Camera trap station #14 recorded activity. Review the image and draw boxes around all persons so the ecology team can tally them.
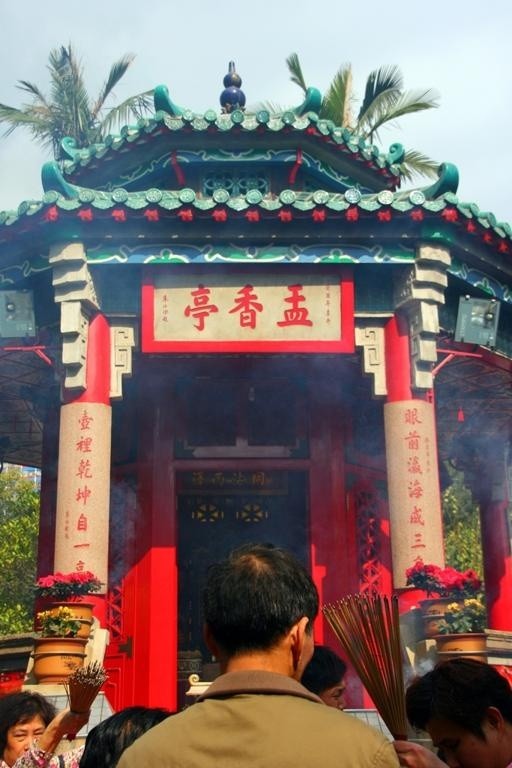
[115,538,399,768]
[300,645,349,713]
[1,689,93,768]
[391,659,511,768]
[78,706,176,767]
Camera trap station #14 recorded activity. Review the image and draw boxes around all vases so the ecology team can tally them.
[44,603,95,639]
[31,637,88,686]
[417,598,464,639]
[433,633,488,666]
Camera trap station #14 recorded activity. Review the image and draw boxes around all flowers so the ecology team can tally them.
[437,598,488,634]
[37,605,83,638]
[33,571,106,602]
[405,561,481,602]
